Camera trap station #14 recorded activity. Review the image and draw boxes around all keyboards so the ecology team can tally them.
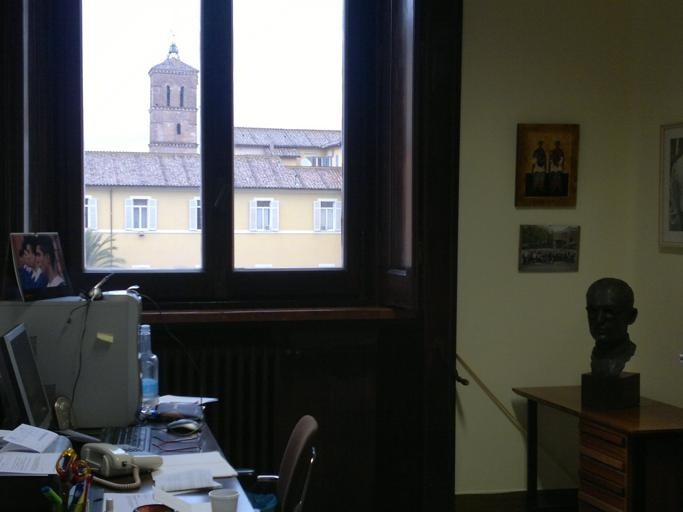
[108,425,153,453]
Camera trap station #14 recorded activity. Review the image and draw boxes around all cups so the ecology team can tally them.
[208,488,241,512]
[133,503,174,511]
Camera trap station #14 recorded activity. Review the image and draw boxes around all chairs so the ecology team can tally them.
[230,411,322,511]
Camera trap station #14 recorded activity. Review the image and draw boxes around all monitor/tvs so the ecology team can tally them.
[2,323,54,429]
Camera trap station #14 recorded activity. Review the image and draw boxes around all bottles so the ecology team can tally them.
[136,324,159,415]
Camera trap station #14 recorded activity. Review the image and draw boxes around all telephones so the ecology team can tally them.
[79,440,164,479]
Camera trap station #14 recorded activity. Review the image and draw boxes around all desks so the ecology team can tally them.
[73,403,259,511]
[508,382,682,512]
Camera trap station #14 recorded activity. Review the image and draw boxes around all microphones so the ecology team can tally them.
[65,298,92,324]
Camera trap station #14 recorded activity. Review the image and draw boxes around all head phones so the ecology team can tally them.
[87,274,118,301]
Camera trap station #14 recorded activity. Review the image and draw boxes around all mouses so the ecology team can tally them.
[167,418,200,435]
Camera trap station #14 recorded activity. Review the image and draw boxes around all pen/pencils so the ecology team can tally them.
[41,474,90,512]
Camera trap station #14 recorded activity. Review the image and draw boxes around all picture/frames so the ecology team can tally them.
[512,121,581,211]
[655,119,682,255]
[8,230,74,304]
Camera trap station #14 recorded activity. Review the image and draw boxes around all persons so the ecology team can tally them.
[17,235,67,289]
[586,277,638,378]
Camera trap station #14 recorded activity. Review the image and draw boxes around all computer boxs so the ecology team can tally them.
[0,289,147,429]
